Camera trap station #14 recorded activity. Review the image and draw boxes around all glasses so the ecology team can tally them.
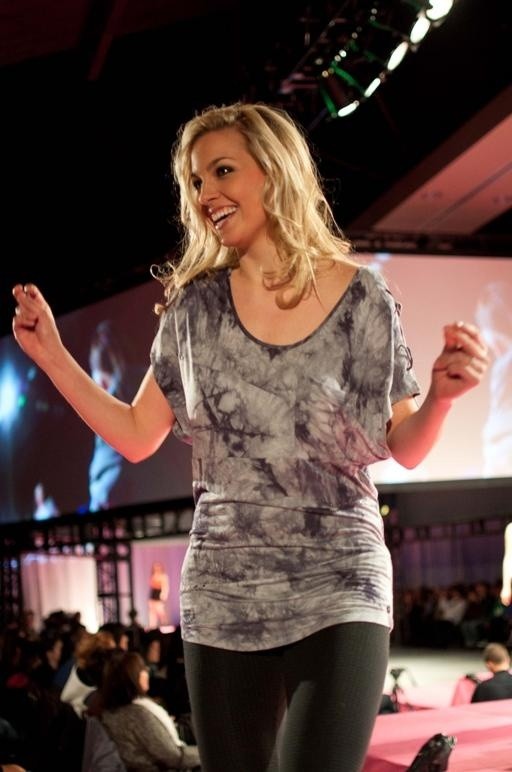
[279,0,454,120]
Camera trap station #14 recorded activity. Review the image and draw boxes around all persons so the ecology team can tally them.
[148,562,169,629]
[471,643,510,703]
[2,102,495,771]
[392,581,510,651]
[1,609,204,770]
